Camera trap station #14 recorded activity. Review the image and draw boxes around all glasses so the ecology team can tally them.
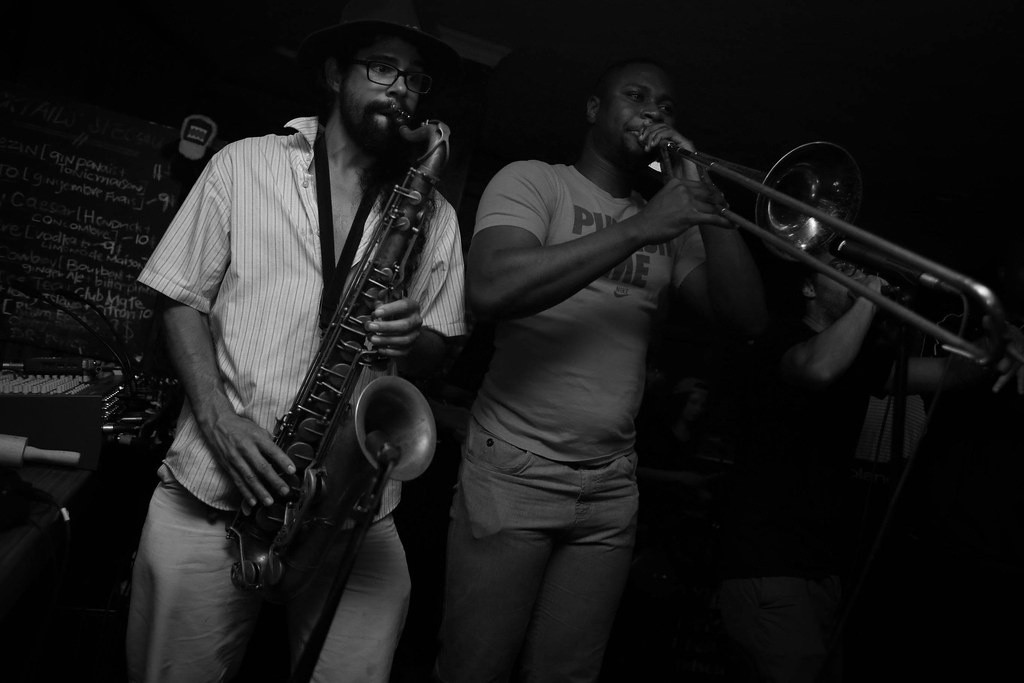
[339,57,432,95]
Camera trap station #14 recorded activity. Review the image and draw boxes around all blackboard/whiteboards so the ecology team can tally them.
[0,82,229,370]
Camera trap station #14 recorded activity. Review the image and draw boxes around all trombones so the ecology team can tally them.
[660,140,1005,369]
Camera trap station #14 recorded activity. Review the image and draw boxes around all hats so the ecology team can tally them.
[296,0,462,100]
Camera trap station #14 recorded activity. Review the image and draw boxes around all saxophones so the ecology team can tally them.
[225,108,457,613]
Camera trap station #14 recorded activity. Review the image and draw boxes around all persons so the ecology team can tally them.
[422,50,767,683]
[650,377,709,456]
[124,0,469,683]
[713,244,1024,683]
